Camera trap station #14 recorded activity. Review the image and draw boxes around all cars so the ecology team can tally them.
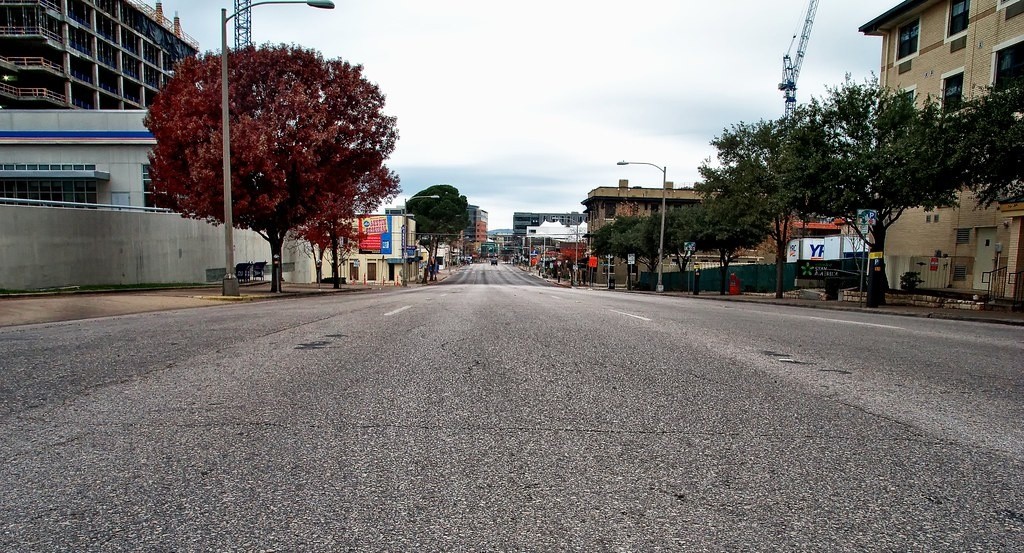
[491,259,497,265]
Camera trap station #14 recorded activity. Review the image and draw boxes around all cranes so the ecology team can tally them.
[778,0,820,121]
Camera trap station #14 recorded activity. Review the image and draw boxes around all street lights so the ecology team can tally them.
[404,195,440,278]
[220,0,336,297]
[617,159,664,292]
[552,217,578,286]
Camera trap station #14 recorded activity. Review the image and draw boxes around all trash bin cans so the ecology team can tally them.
[729,279,740,295]
[610,279,615,289]
[429,264,439,275]
